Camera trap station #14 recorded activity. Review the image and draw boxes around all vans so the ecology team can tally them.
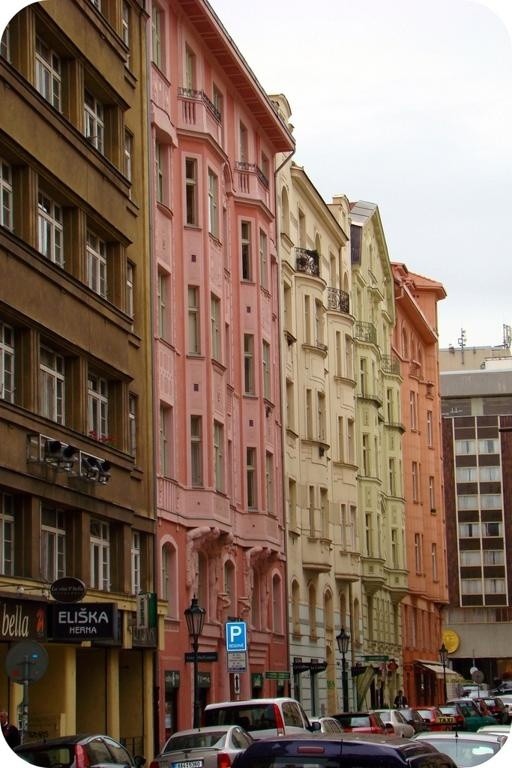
[203,696,321,740]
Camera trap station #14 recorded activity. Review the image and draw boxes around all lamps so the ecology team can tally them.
[71,450,111,485]
[26,432,78,474]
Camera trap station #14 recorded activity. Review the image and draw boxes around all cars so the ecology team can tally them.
[16,734,146,768]
[313,693,511,739]
[148,725,252,768]
[232,725,509,767]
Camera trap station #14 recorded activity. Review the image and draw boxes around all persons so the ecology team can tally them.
[0,711,18,748]
[393,690,408,709]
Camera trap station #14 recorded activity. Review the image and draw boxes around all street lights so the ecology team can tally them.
[185,593,206,729]
[337,625,351,712]
[440,642,449,704]
